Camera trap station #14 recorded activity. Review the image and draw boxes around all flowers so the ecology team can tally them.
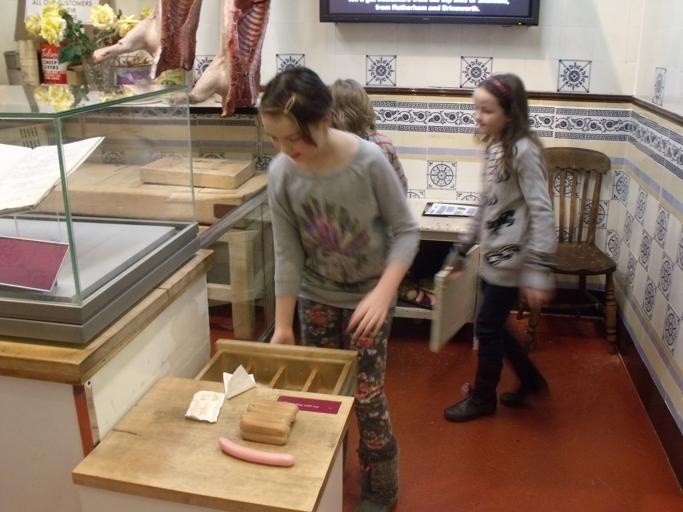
[21,0,158,68]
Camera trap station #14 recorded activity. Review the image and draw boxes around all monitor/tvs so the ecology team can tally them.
[320,0,540,26]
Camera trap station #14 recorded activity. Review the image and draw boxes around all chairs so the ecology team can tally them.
[515,145,620,356]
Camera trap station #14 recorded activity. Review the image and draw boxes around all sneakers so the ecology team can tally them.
[354,496,390,512]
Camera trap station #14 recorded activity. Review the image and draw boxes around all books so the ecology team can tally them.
[427,241,481,356]
[0,134,109,223]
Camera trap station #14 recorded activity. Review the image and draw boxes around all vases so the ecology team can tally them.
[80,53,118,87]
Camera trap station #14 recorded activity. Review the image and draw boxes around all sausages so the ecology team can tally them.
[240,400,298,445]
[220,437,293,467]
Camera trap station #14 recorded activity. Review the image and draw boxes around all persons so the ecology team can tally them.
[256,64,427,512]
[322,78,407,206]
[440,68,563,424]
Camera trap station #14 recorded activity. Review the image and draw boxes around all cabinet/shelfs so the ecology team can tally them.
[0,82,198,324]
[390,198,482,351]
[70,337,364,510]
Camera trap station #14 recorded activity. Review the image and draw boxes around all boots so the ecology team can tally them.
[500,362,549,410]
[443,376,500,424]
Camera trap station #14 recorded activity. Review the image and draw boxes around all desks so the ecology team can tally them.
[0,248,221,511]
[195,208,275,340]
